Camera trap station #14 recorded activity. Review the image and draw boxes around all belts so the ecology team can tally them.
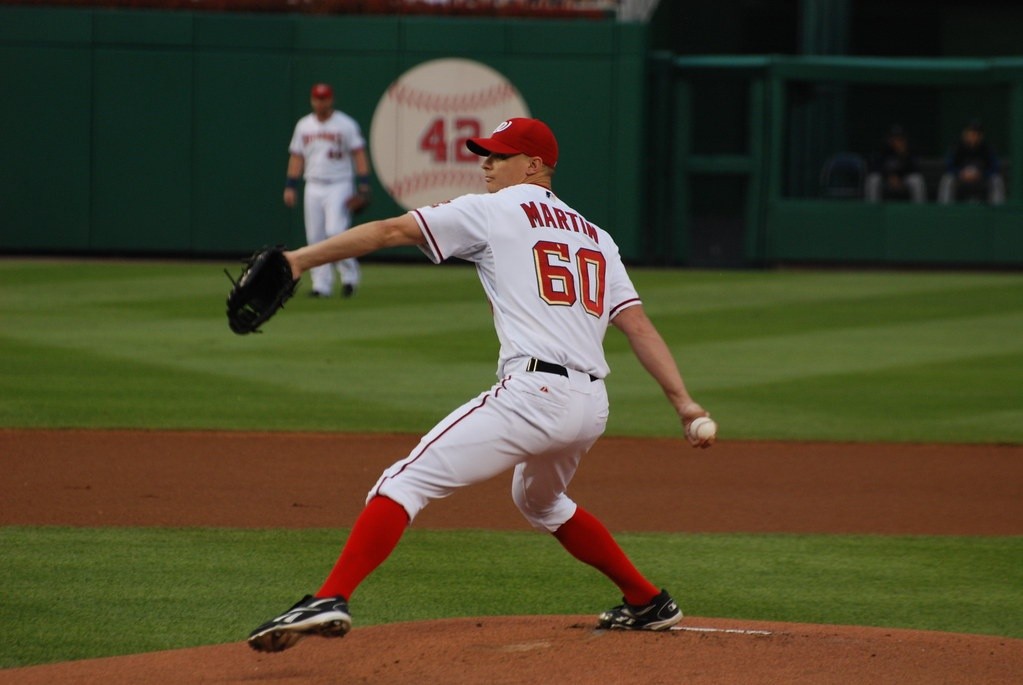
[526,358,599,382]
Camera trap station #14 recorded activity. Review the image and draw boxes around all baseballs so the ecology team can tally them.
[687,415,718,442]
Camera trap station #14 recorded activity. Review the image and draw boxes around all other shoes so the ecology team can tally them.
[308,291,319,298]
[341,284,352,298]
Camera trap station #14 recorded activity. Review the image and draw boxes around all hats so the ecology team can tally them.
[311,83,331,98]
[466,117,559,169]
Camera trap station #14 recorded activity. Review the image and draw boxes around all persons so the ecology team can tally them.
[864,131,924,205]
[283,83,373,299]
[939,126,1006,207]
[226,118,715,655]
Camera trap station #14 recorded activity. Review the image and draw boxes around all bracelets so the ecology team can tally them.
[357,177,369,183]
[284,177,295,188]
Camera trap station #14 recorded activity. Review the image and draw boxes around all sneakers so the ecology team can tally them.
[248,595,351,653]
[599,589,684,631]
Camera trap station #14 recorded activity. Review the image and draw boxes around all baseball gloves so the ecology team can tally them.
[226,242,302,336]
